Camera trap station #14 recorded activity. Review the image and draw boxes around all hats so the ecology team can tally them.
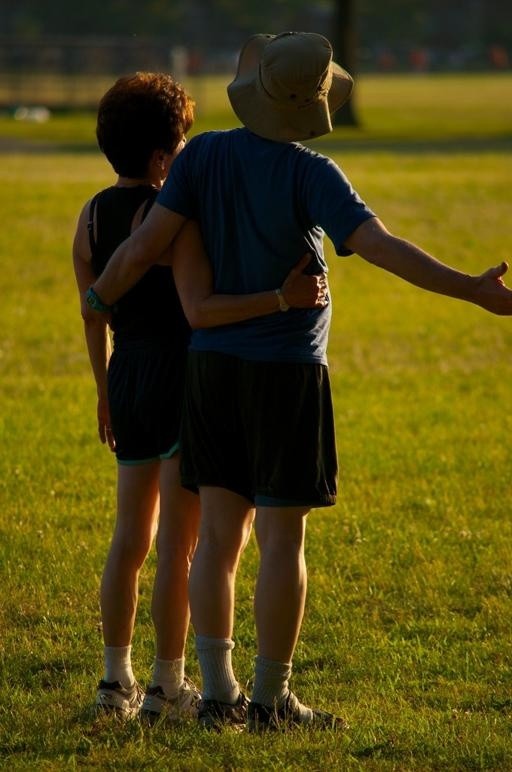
[228,32,354,141]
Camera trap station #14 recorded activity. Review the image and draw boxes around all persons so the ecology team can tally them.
[73,73,327,730]
[82,32,512,732]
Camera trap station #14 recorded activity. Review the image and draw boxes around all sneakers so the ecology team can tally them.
[96,676,345,734]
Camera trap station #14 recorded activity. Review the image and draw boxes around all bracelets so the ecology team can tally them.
[85,287,113,312]
[275,287,290,313]
